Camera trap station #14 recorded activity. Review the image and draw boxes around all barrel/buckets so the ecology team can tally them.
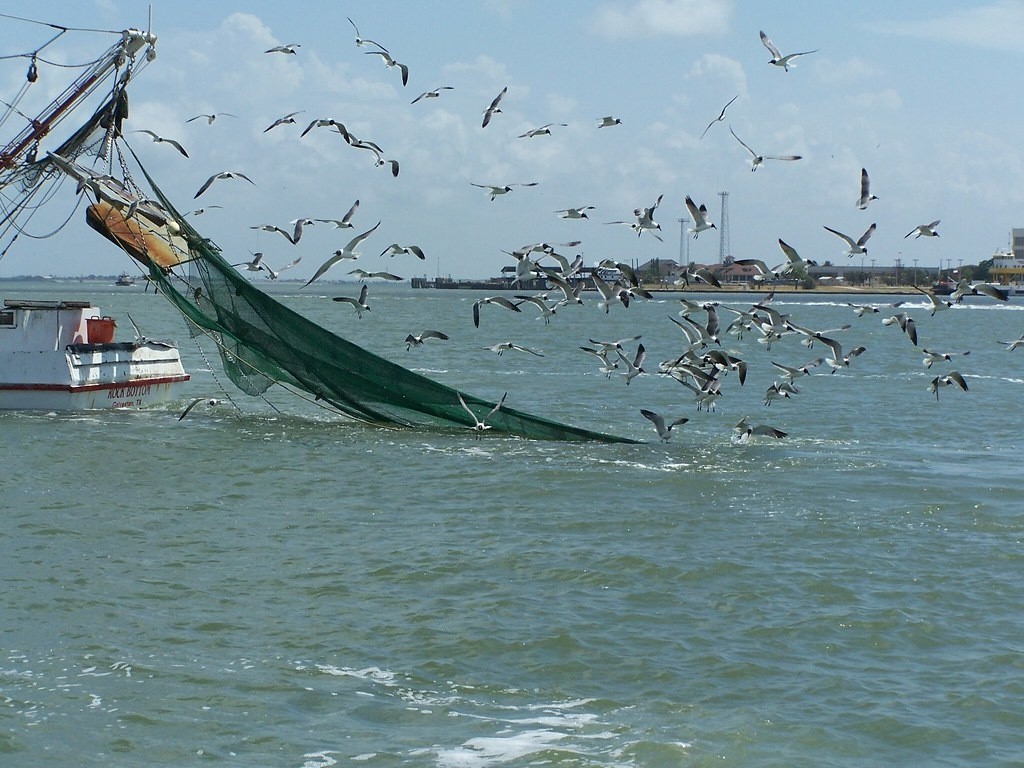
[86,316,116,343]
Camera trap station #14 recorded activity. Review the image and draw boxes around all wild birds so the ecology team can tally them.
[124,15,1024,446]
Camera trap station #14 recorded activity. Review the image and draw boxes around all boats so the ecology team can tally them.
[489,266,633,291]
[115,275,134,286]
[0,299,190,409]
